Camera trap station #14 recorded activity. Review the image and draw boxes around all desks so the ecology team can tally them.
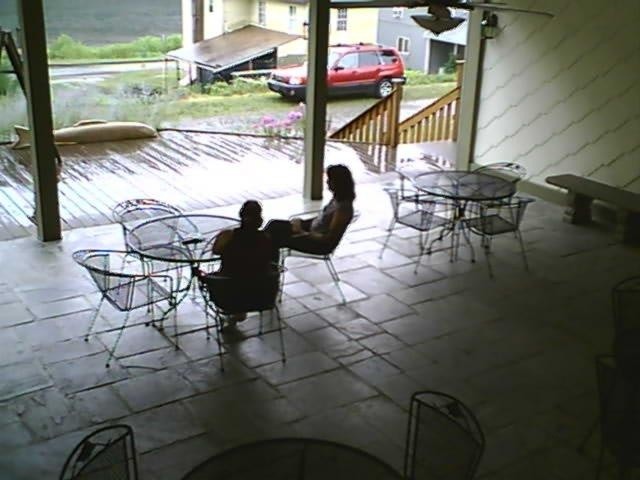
[127,212,242,340]
[182,437,404,480]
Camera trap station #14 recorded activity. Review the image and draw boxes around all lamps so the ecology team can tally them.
[411,4,467,36]
[481,14,501,40]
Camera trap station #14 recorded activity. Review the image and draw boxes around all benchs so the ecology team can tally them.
[545,174,640,242]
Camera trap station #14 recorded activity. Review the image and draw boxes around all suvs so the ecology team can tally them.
[268,41,407,100]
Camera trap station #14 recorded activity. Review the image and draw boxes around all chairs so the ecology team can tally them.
[577,277,640,480]
[59,423,138,479]
[73,249,179,370]
[380,162,536,277]
[112,198,204,328]
[276,209,360,303]
[195,264,289,371]
[404,390,486,480]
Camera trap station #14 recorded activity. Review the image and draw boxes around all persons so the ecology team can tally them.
[262,163,357,256]
[211,199,273,323]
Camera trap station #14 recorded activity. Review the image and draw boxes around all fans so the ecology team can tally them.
[333,0,555,17]
